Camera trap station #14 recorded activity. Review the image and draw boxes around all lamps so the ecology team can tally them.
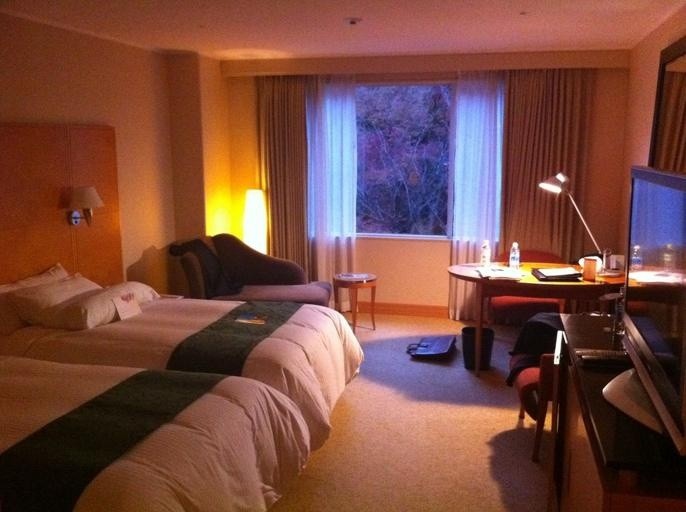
[537,172,603,255]
[67,187,104,227]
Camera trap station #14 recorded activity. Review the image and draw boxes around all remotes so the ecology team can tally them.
[575,349,629,362]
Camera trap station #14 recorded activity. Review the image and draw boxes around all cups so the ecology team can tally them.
[583,258,597,283]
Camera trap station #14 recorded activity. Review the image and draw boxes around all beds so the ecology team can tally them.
[1,355,310,511]
[1,291,365,453]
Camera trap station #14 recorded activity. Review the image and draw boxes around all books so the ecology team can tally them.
[538,266,581,277]
[477,264,522,282]
[531,267,581,282]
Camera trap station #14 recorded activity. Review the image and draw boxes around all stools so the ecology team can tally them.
[333,272,376,336]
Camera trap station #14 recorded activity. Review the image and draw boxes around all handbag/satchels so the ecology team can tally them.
[407,335,456,358]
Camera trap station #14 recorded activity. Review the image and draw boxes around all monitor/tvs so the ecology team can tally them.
[600,163,686,459]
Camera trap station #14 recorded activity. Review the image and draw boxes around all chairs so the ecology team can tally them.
[167,233,332,308]
[508,311,560,463]
[486,250,568,322]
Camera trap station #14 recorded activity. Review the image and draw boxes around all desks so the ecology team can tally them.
[446,261,626,381]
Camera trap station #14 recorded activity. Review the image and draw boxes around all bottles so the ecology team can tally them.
[613,287,627,337]
[662,244,675,273]
[480,239,491,271]
[508,242,520,272]
[630,245,644,272]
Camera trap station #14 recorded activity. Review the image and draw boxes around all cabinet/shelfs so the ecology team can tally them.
[559,313,684,511]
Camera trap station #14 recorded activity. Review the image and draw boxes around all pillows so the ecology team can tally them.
[0,262,160,339]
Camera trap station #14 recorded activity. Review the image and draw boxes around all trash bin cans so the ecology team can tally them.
[462,327,494,370]
